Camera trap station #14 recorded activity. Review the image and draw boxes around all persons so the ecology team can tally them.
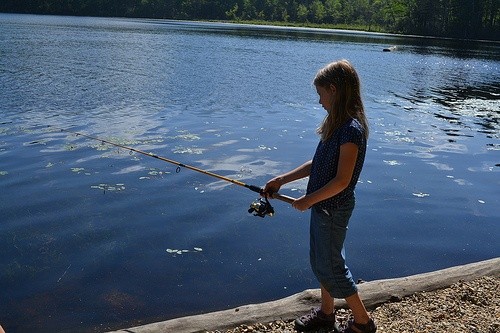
[260,57,382,333]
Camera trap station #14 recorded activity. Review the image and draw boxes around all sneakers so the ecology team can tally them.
[343,318,376,333]
[295,307,335,330]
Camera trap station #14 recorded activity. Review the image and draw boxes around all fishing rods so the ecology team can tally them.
[34,123,312,211]
